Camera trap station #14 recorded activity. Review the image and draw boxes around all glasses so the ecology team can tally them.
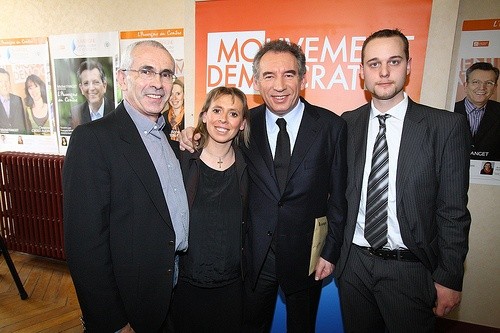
[82,81,102,88]
[467,80,495,87]
[121,68,178,84]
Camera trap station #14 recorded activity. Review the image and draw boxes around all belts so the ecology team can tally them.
[364,246,421,262]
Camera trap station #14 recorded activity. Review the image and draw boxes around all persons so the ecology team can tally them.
[161,79,185,141]
[24,74,55,135]
[0,68,26,134]
[62,137,67,146]
[180,41,347,333]
[174,87,248,333]
[18,136,23,144]
[454,62,500,161]
[334,29,471,333]
[1,135,5,142]
[70,60,115,130]
[60,41,192,333]
[480,162,493,175]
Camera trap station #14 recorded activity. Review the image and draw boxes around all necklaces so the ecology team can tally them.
[217,158,223,168]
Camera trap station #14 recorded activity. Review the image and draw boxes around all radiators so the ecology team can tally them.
[0,151,67,261]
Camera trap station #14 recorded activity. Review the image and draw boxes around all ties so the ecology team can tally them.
[364,114,392,250]
[274,118,291,197]
